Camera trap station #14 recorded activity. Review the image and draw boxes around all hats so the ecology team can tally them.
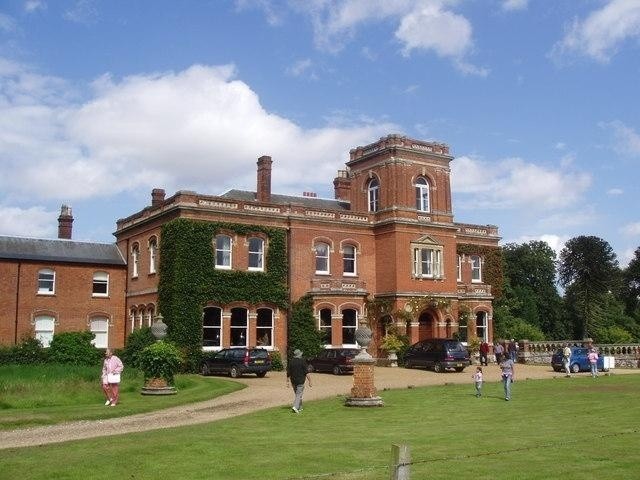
[292,348,304,359]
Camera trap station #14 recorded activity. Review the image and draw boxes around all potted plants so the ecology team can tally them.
[379,334,404,368]
[470,336,482,367]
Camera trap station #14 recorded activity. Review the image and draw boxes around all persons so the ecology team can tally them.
[562,342,571,378]
[287,349,312,413]
[588,346,599,379]
[101,349,124,406]
[473,338,519,401]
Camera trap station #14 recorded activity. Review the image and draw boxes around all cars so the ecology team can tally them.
[404,338,471,374]
[306,348,362,374]
[549,348,609,376]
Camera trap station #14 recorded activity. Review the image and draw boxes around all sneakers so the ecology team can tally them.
[110,403,116,407]
[104,398,111,406]
[292,406,304,414]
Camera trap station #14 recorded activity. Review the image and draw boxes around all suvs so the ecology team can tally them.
[200,347,271,378]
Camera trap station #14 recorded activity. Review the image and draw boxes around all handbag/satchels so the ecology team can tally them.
[106,370,121,384]
